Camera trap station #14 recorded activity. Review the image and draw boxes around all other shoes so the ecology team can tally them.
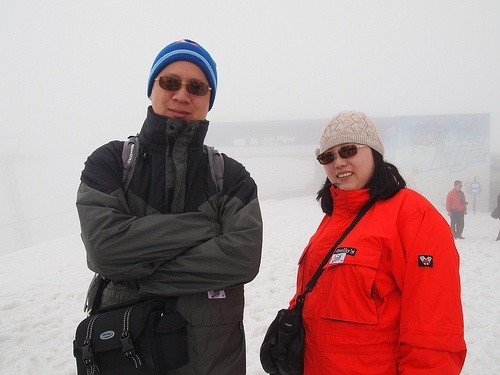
[454,235,465,239]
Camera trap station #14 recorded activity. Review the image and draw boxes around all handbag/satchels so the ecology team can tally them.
[260,308,305,375]
[72,295,189,375]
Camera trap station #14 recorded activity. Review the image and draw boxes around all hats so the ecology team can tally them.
[147,38,217,112]
[314,110,384,157]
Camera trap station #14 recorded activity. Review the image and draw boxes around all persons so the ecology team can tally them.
[446,181,467,239]
[288,111,466,375]
[77,39,262,375]
[496,193,500,240]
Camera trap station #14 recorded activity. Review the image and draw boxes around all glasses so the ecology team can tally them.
[316,144,368,165]
[154,75,212,96]
